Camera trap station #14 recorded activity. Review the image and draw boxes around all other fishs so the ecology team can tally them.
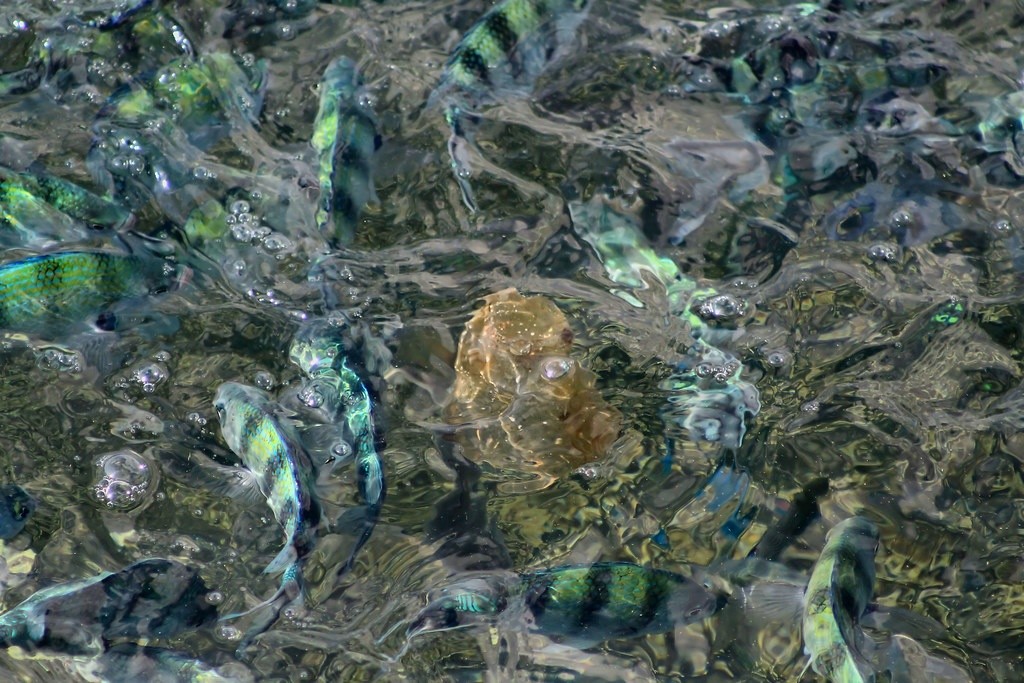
[0,1,1024,683]
